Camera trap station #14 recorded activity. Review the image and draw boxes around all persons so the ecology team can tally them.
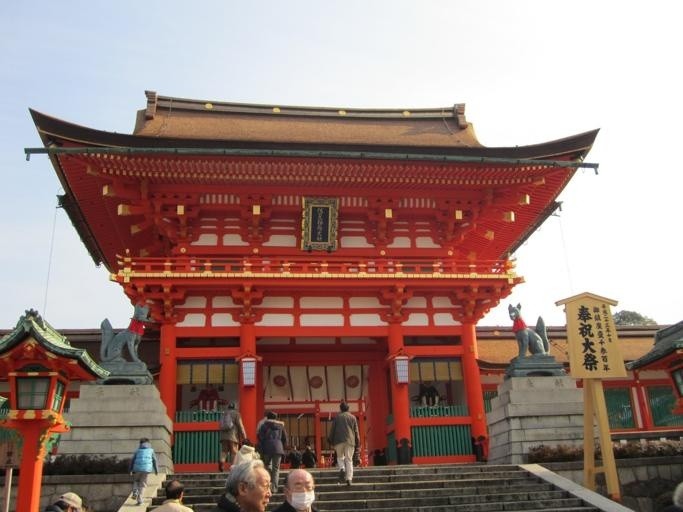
[211,461,316,512]
[218,401,286,493]
[150,480,194,512]
[41,491,82,512]
[328,401,358,486]
[287,445,317,470]
[128,437,159,503]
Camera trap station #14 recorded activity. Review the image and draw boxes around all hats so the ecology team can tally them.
[59,492,82,512]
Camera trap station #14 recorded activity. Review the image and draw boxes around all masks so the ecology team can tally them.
[287,490,316,510]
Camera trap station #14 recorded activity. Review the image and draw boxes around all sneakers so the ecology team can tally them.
[131,491,144,506]
[219,460,224,472]
[339,470,352,486]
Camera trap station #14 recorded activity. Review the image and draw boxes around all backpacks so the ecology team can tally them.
[219,410,234,432]
